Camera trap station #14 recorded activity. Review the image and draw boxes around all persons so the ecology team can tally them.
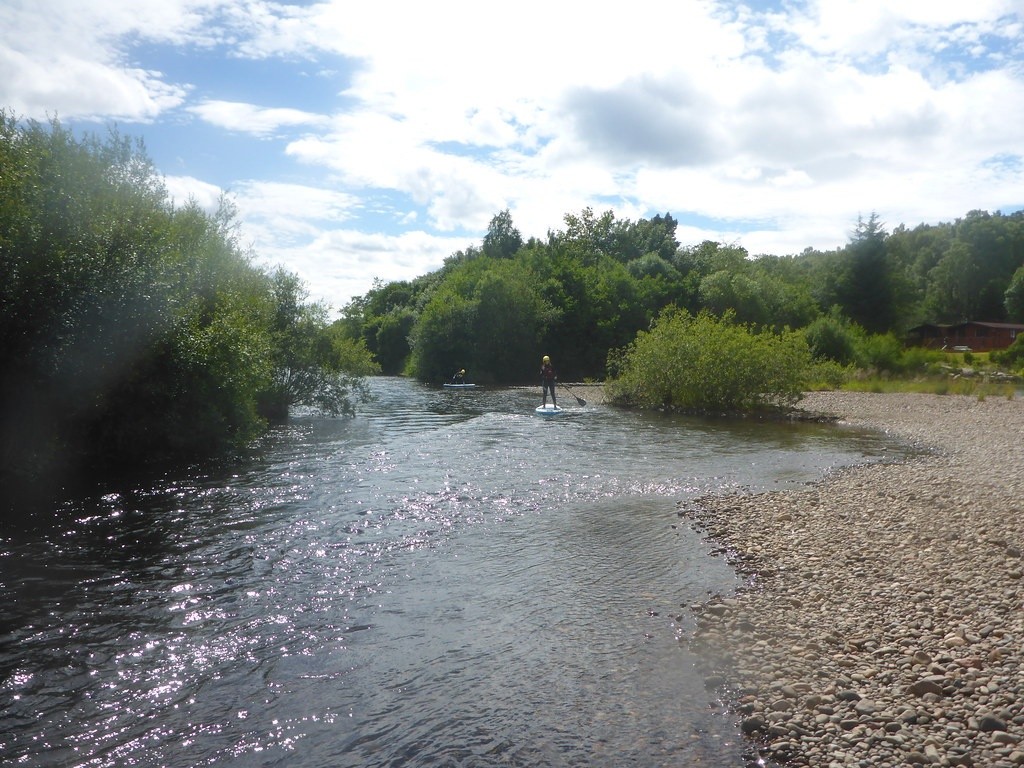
[450,368,466,384]
[538,356,561,410]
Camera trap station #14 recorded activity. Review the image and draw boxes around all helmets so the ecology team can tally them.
[543,356,550,363]
[461,370,465,374]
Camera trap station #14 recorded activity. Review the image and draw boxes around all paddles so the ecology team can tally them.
[557,379,586,407]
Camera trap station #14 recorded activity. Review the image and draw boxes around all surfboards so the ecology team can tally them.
[536,404,562,415]
[444,384,475,387]
